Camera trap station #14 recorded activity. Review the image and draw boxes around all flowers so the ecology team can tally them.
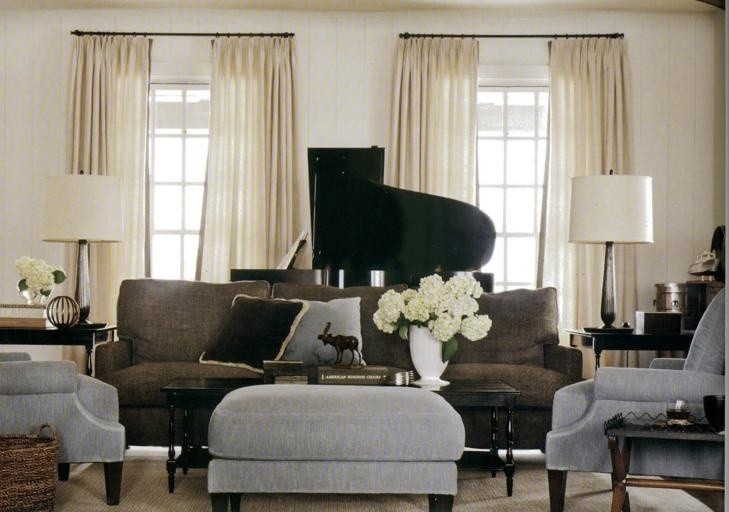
[14,255,67,297]
[373,274,493,363]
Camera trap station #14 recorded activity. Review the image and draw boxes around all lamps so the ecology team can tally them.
[39,170,124,329]
[568,169,654,332]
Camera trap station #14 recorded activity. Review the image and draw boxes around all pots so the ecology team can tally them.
[653,283,688,313]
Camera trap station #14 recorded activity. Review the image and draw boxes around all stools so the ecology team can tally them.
[207,383,465,512]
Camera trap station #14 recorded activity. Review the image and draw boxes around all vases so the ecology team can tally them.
[21,291,47,305]
[409,325,450,385]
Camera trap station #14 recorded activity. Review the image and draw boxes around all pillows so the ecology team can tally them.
[284,297,367,367]
[199,293,309,374]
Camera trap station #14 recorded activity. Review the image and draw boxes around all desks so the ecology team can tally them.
[0,318,117,375]
[565,325,695,369]
[605,417,725,512]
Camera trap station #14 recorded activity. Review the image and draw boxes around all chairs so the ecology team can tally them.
[0,351,125,506]
[544,285,725,511]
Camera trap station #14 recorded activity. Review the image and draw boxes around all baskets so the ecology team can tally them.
[0,424,61,512]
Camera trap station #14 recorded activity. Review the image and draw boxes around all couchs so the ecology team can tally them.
[94,279,583,454]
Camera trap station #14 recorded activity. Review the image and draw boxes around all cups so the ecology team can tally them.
[703,395,725,430]
[667,397,691,426]
